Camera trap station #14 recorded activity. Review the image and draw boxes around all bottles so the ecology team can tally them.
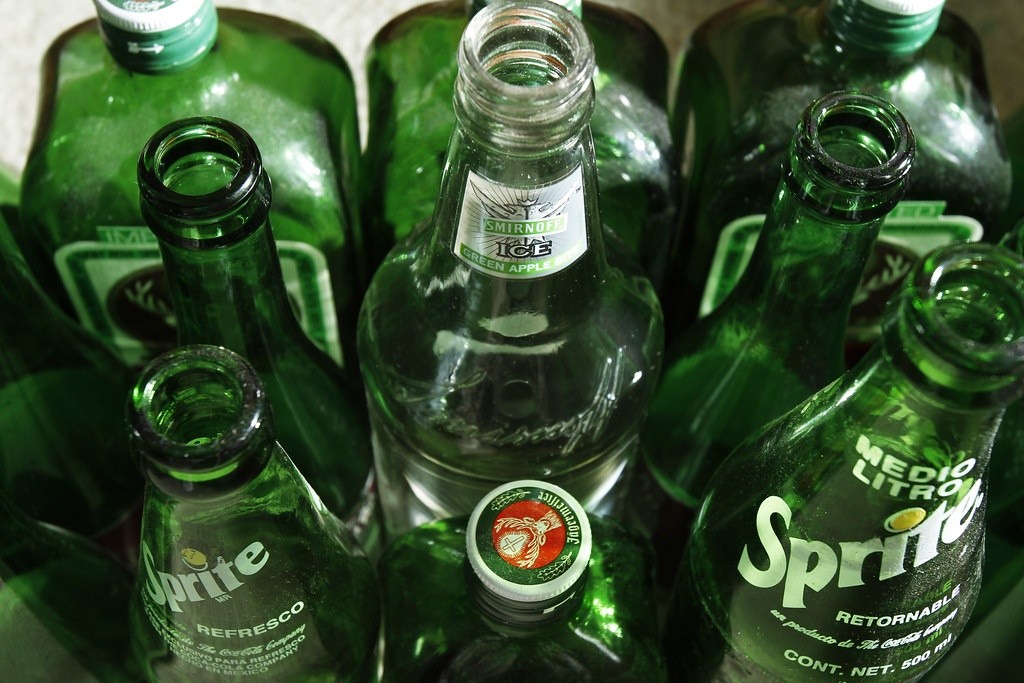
[673,0,1012,367]
[357,0,678,293]
[20,0,362,382]
[0,497,124,683]
[382,479,668,683]
[0,229,137,574]
[362,0,664,536]
[622,90,914,629]
[670,244,1024,683]
[125,345,378,683]
[138,118,385,561]
